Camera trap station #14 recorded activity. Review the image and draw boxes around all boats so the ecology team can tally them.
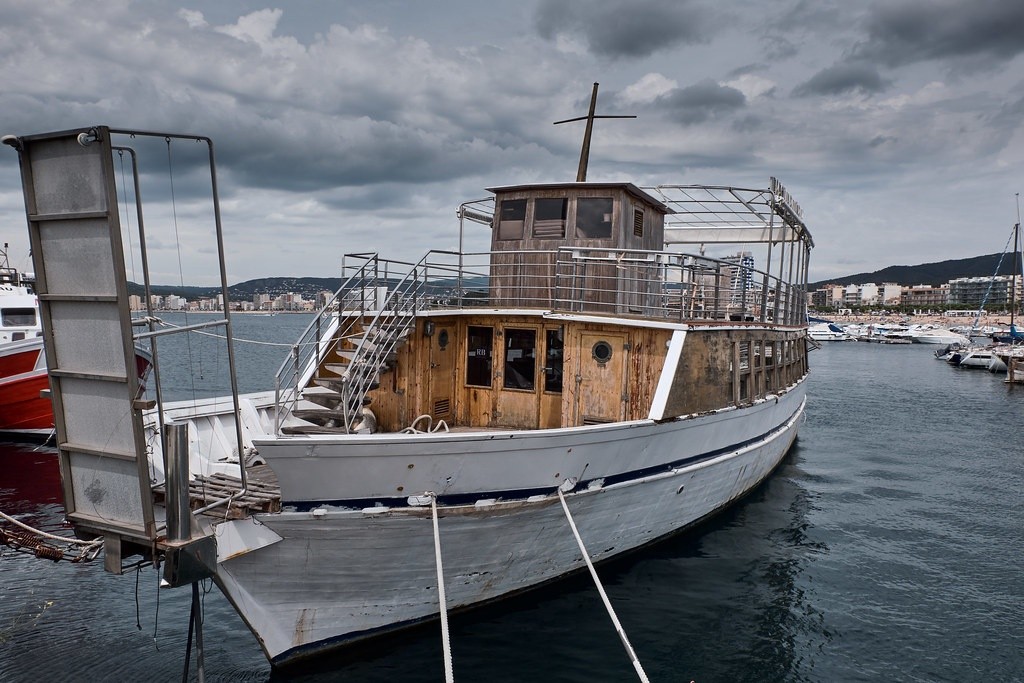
[0,244,155,437]
[169,82,815,670]
[806,191,1024,384]
